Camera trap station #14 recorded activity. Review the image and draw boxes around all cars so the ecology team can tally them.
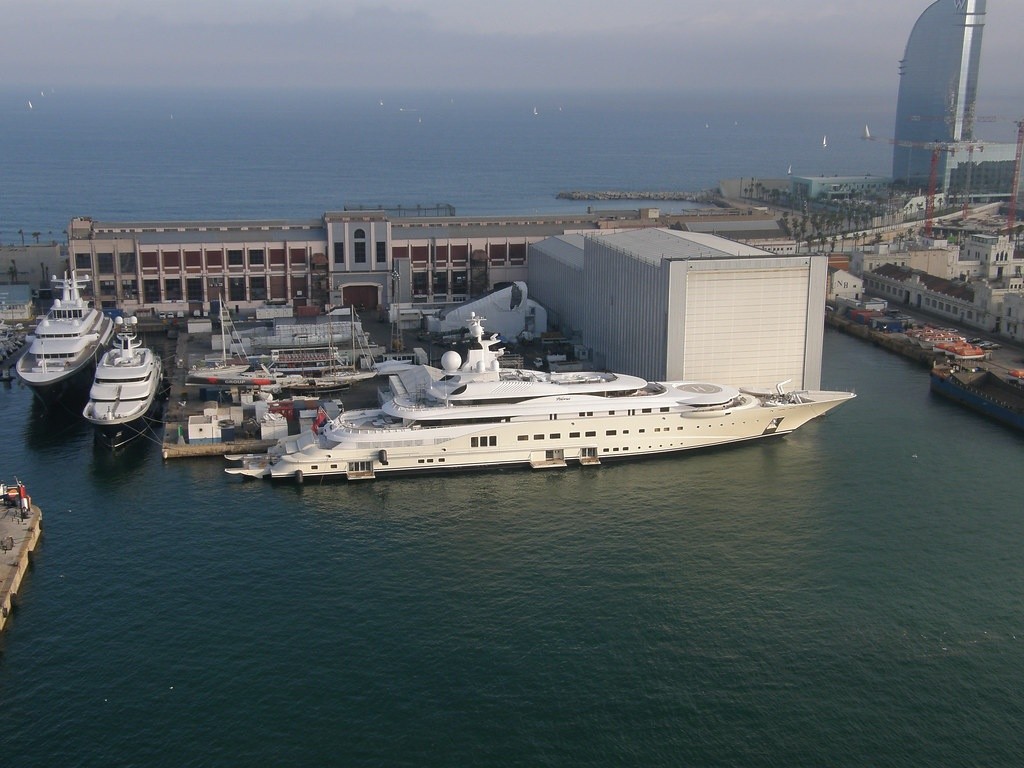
[985,343,1002,350]
[976,340,994,348]
[967,338,982,344]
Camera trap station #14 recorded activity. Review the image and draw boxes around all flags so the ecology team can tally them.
[311,409,326,433]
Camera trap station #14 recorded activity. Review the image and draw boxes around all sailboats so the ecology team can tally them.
[181,287,419,394]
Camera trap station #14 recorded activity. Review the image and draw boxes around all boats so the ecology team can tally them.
[954,346,985,360]
[13,267,118,410]
[1007,367,1024,387]
[918,330,967,350]
[932,339,966,353]
[223,307,859,483]
[904,326,942,345]
[944,339,973,356]
[82,314,165,449]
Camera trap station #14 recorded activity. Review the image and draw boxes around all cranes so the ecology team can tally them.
[852,103,1024,244]
